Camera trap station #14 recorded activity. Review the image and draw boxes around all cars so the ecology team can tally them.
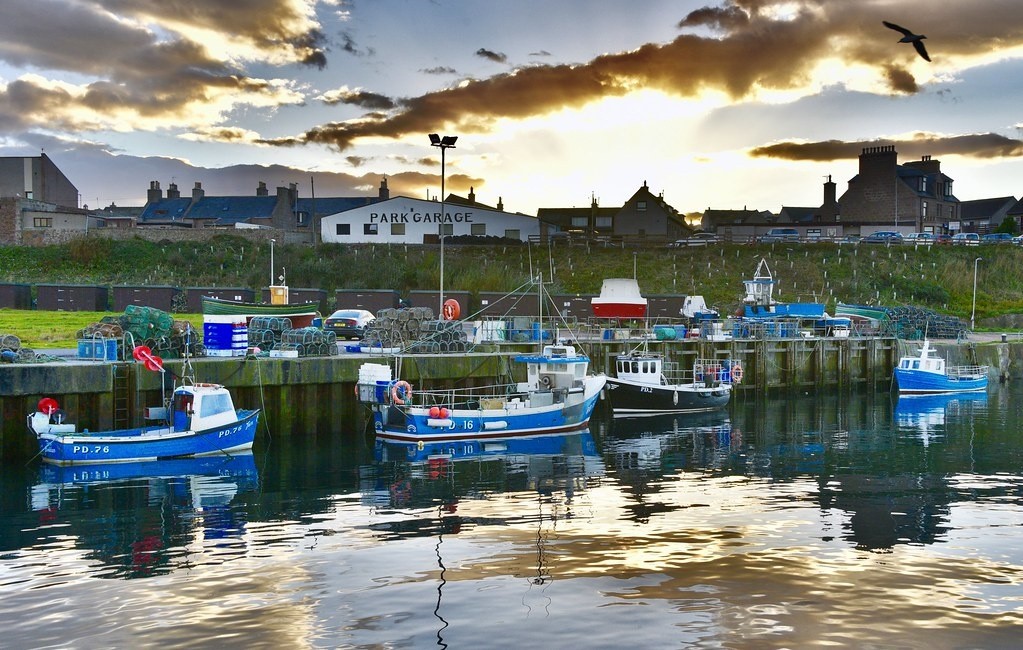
[323,309,375,339]
[860,231,904,245]
[952,233,980,247]
[755,229,801,243]
[675,233,724,246]
[903,233,934,245]
[982,233,1023,245]
[931,234,953,245]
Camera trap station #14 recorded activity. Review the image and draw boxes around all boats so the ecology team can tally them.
[25,342,262,467]
[742,280,825,318]
[893,341,989,394]
[201,285,321,315]
[591,278,647,321]
[604,356,744,418]
[678,295,720,323]
[353,271,605,444]
[835,302,891,335]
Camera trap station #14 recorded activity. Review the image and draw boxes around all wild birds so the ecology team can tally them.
[882,20,932,63]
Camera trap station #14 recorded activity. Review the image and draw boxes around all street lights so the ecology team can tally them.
[428,133,458,322]
[972,258,983,329]
[270,239,276,286]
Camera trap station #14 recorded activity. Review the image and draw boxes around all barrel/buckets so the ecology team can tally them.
[656,328,677,340]
[76,305,207,361]
[247,316,339,358]
[359,306,475,354]
[0,334,35,361]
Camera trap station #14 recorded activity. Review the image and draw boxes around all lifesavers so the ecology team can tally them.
[194,383,218,387]
[354,382,358,396]
[444,304,455,320]
[731,366,743,382]
[392,380,412,404]
